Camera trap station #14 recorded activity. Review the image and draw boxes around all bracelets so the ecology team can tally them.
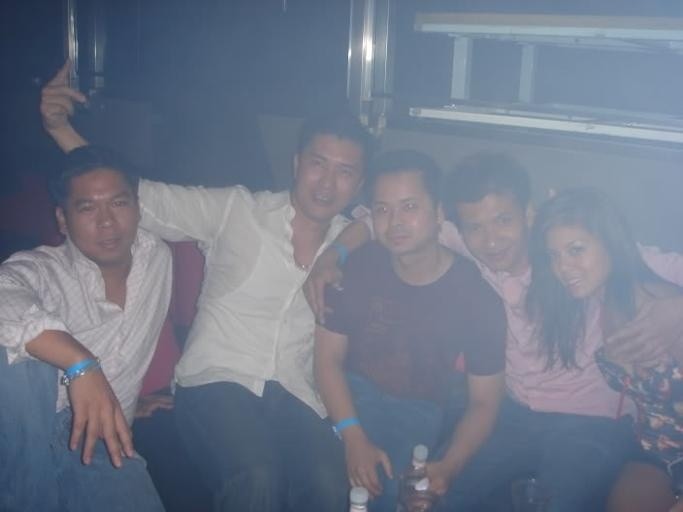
[333,417,360,436]
[63,359,100,378]
[61,357,101,387]
[325,242,347,268]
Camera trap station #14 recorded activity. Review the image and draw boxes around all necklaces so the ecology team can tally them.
[293,258,310,270]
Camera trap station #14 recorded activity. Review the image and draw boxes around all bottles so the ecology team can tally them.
[393,443,428,512]
[346,485,370,512]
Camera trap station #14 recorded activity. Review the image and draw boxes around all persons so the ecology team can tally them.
[520,188,683,512]
[38,57,373,510]
[311,150,507,512]
[0,143,182,510]
[302,149,682,512]
